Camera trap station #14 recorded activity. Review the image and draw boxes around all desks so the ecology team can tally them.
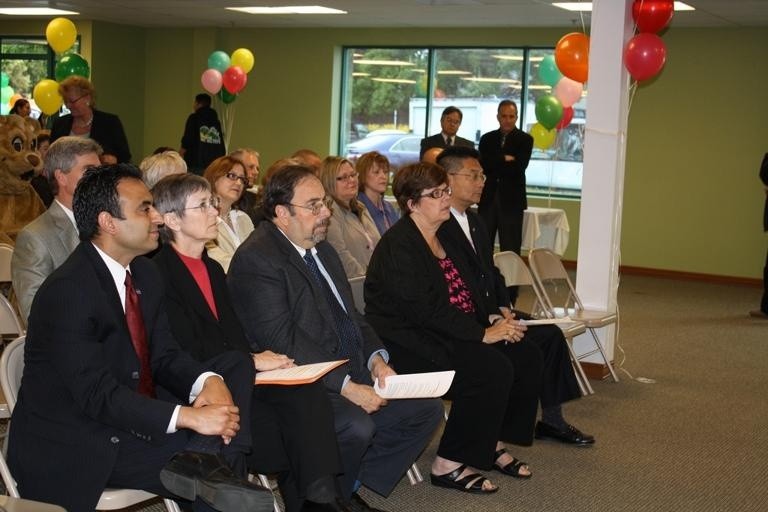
[388,192,571,259]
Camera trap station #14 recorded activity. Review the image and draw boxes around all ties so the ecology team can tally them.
[303,248,361,383]
[446,136,452,148]
[123,269,160,401]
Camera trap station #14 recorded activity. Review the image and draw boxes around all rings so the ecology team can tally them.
[506,329,510,335]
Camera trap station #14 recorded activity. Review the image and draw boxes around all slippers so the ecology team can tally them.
[493,449,532,478]
[430,463,500,494]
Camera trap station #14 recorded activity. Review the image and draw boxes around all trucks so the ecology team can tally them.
[406,96,545,150]
[522,95,588,202]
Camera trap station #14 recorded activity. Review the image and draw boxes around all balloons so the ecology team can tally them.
[623,33,666,84]
[631,0,674,34]
[201,48,255,104]
[0,17,92,117]
[528,32,588,152]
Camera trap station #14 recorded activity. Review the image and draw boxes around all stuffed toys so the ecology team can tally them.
[0,114,47,248]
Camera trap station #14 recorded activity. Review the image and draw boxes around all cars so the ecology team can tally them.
[346,134,432,186]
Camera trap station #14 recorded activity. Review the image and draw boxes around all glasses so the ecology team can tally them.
[417,186,452,200]
[449,173,487,185]
[336,172,360,183]
[172,197,221,214]
[226,172,250,187]
[284,197,334,216]
[63,96,85,107]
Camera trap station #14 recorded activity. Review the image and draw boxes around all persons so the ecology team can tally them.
[476,101,534,255]
[419,105,474,164]
[363,160,532,495]
[49,77,132,164]
[6,164,275,511]
[9,99,31,116]
[1,150,397,328]
[749,151,767,320]
[436,146,595,447]
[129,173,384,512]
[181,93,226,178]
[220,167,446,499]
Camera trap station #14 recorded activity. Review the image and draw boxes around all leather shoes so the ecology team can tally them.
[298,499,355,512]
[535,420,595,446]
[750,310,768,319]
[337,491,386,512]
[159,449,275,512]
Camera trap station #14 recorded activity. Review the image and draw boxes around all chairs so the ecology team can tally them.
[0,243,16,290]
[0,290,22,342]
[491,250,586,399]
[0,451,63,512]
[528,246,620,393]
[2,334,177,512]
[346,275,425,482]
